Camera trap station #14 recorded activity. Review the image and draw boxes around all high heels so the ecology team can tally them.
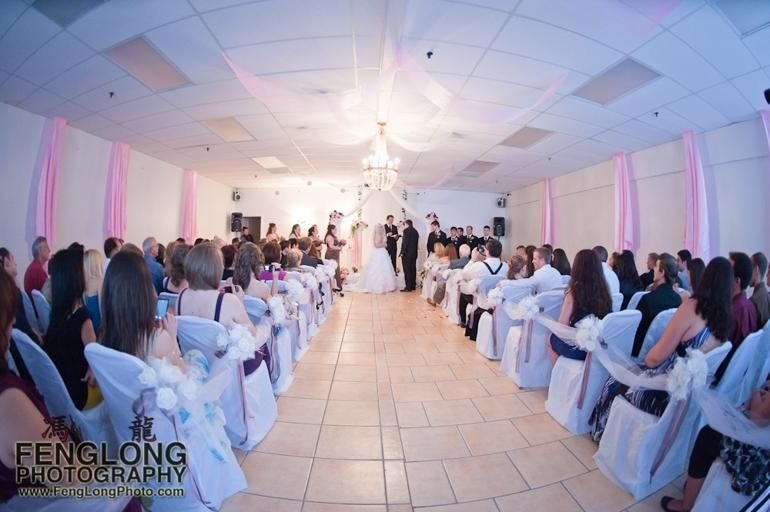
[333,288,340,292]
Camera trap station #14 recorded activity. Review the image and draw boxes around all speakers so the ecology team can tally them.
[493,217,505,235]
[230,212,243,232]
[233,191,241,200]
[496,197,505,208]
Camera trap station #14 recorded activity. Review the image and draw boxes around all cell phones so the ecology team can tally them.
[154,297,169,323]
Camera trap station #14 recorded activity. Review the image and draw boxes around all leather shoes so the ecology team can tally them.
[427,298,436,307]
[400,288,411,291]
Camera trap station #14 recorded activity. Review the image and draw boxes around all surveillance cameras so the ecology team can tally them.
[506,192,510,198]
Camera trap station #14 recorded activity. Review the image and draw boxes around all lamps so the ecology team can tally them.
[361,117,403,192]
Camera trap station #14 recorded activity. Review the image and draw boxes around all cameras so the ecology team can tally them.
[477,247,484,253]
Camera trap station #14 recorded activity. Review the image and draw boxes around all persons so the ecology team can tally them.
[400,219,419,292]
[0,224,346,511]
[368,223,397,293]
[423,221,769,512]
[382,214,398,272]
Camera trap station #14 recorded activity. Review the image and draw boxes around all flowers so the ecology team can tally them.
[265,206,493,259]
